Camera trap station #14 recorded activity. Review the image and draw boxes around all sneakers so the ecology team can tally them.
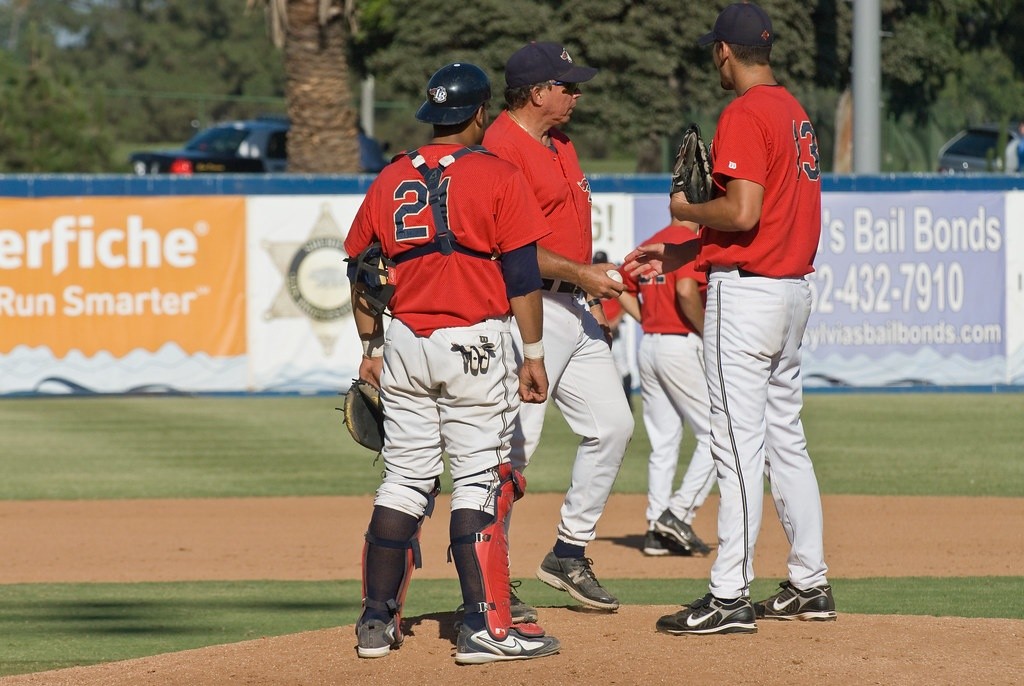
[656,592,758,634]
[655,508,709,556]
[536,547,620,610]
[644,530,692,556]
[509,580,538,623]
[455,623,561,664]
[752,580,837,620]
[357,617,394,657]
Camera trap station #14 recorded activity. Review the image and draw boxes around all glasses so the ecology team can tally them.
[550,81,577,87]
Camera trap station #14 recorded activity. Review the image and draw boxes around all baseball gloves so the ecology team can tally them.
[669,122,712,205]
[343,378,385,453]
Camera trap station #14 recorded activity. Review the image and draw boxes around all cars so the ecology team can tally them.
[126,118,392,175]
[936,123,1022,174]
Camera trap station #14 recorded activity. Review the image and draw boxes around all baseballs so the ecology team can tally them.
[605,269,623,283]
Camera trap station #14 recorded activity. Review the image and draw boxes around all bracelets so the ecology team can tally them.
[523,339,545,359]
[363,335,385,357]
[588,299,601,306]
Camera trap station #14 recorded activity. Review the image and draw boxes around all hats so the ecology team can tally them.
[696,2,774,45]
[506,41,598,87]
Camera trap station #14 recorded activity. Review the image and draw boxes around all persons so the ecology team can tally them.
[483,43,630,626]
[618,215,718,555]
[347,62,558,663]
[594,251,631,408]
[624,2,835,635]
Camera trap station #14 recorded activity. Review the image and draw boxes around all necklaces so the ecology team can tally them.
[508,111,528,132]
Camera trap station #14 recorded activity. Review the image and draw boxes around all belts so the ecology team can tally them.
[661,333,688,336]
[541,278,581,294]
[738,268,763,277]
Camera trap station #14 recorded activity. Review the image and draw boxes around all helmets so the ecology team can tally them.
[414,63,490,125]
